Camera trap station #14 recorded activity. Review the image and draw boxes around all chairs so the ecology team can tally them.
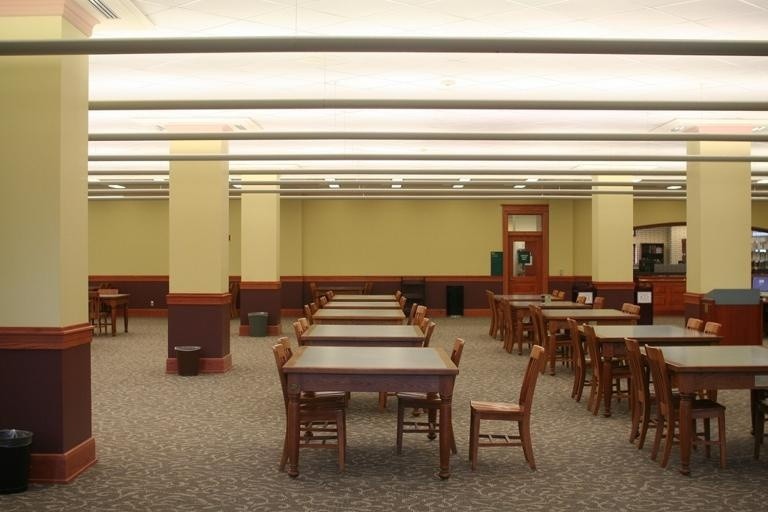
[401,298,407,309]
[298,318,350,399]
[310,302,316,314]
[323,296,327,304]
[364,283,368,294]
[580,296,586,304]
[88,291,111,336]
[277,337,347,443]
[326,292,331,302]
[400,296,405,305]
[528,305,566,366]
[501,297,530,349]
[558,291,565,298]
[397,337,465,454]
[754,400,768,460]
[751,387,768,436]
[380,321,435,410]
[420,317,429,334]
[584,326,632,416]
[535,306,574,374]
[293,322,348,408]
[624,339,696,450]
[469,345,544,473]
[304,305,312,325]
[396,292,401,301]
[313,282,316,294]
[412,306,427,326]
[407,303,417,325]
[699,322,722,400]
[98,289,118,294]
[330,290,333,297]
[396,290,400,297]
[368,282,373,294]
[623,303,633,313]
[645,344,726,469]
[577,295,581,303]
[504,298,534,353]
[610,305,640,367]
[320,298,325,308]
[486,289,494,336]
[488,291,505,339]
[567,318,620,403]
[553,290,558,296]
[310,283,313,293]
[583,297,604,354]
[272,344,344,473]
[646,318,705,399]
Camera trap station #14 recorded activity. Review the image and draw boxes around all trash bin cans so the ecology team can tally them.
[174,346,201,377]
[248,311,268,337]
[0,429,33,495]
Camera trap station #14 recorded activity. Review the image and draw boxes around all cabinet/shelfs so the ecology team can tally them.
[638,276,686,315]
[401,276,426,317]
[751,235,768,275]
[701,299,763,345]
